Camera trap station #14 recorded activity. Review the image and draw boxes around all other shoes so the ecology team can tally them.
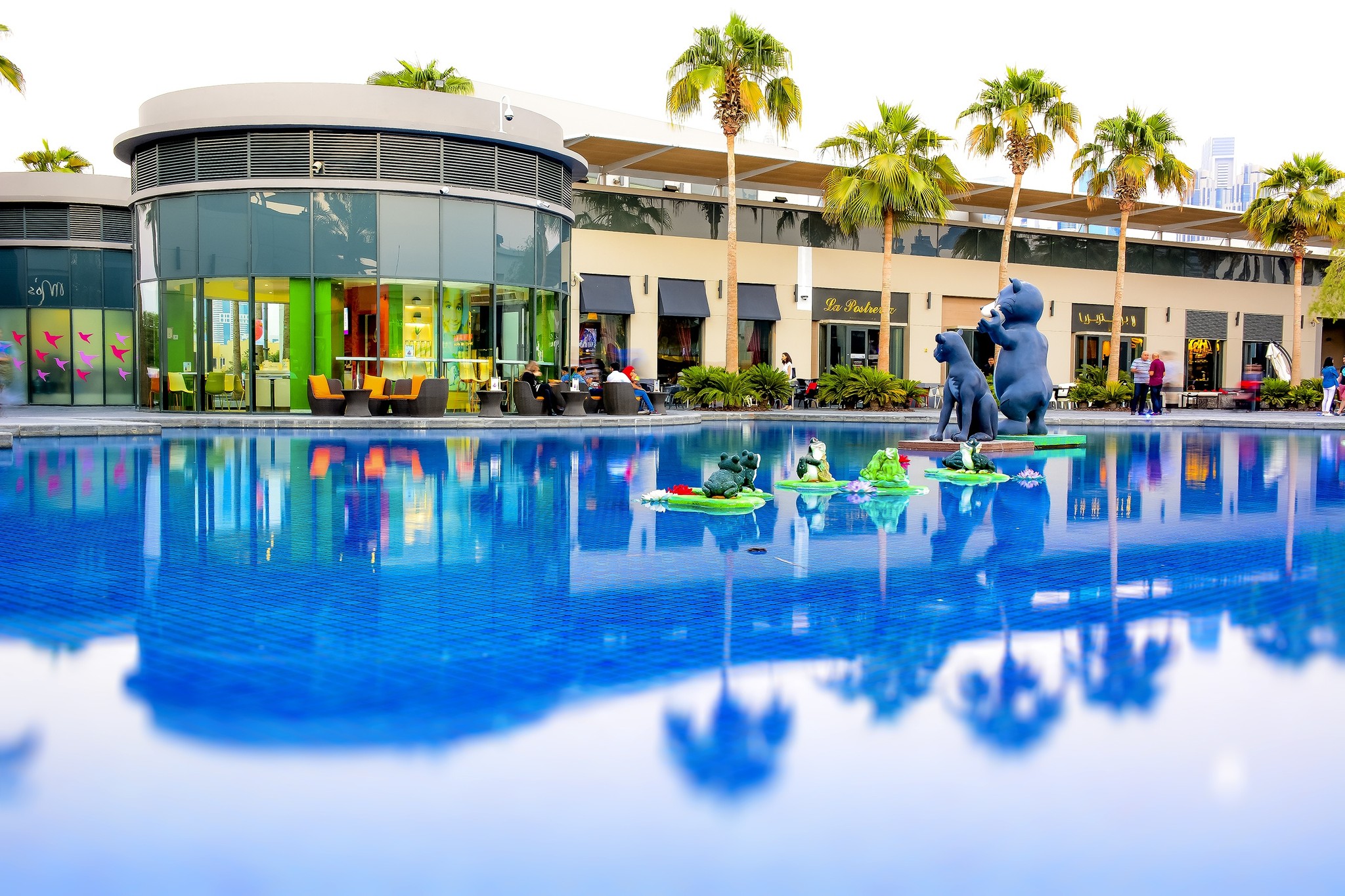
[1341,413,1345,416]
[1131,411,1135,415]
[780,407,788,410]
[557,407,565,411]
[650,411,662,416]
[1149,411,1162,415]
[786,407,793,410]
[1138,411,1145,415]
[1322,413,1334,416]
[548,413,557,416]
[638,410,649,415]
[1333,413,1340,416]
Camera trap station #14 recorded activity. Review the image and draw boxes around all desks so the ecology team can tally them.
[340,388,372,417]
[179,372,210,411]
[560,391,589,417]
[646,392,670,415]
[476,390,507,418]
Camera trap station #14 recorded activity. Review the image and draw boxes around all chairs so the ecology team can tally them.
[770,378,845,409]
[167,372,194,412]
[405,361,429,380]
[381,360,406,382]
[194,372,226,413]
[229,374,246,410]
[214,373,239,410]
[514,363,527,413]
[638,382,714,410]
[146,367,171,411]
[471,355,499,413]
[1048,382,1079,410]
[452,359,489,413]
[909,384,958,410]
[486,356,510,413]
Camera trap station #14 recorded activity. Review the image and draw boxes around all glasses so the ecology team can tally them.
[609,367,611,369]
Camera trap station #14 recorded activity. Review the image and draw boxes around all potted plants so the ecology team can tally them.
[412,311,422,323]
[415,326,423,340]
[412,297,422,305]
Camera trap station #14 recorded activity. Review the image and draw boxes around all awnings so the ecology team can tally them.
[658,277,710,317]
[737,284,781,321]
[578,273,635,313]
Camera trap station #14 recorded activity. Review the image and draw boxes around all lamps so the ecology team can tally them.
[1235,311,1240,326]
[1300,315,1304,329]
[717,280,722,298]
[1166,307,1170,322]
[794,284,798,302]
[643,275,648,294]
[927,292,931,309]
[1050,301,1054,316]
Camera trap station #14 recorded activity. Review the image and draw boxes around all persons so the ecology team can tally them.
[607,362,662,415]
[1147,351,1166,415]
[664,371,687,402]
[572,366,605,413]
[1333,355,1345,416]
[781,352,796,409]
[984,356,994,376]
[561,366,570,383]
[623,365,642,387]
[520,361,565,416]
[443,288,464,337]
[1130,350,1153,415]
[1321,356,1340,416]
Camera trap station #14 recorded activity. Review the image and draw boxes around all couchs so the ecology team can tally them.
[511,380,549,416]
[352,377,393,417]
[1147,390,1246,410]
[602,381,641,415]
[390,378,414,417]
[407,378,449,418]
[306,374,348,417]
[565,381,603,414]
